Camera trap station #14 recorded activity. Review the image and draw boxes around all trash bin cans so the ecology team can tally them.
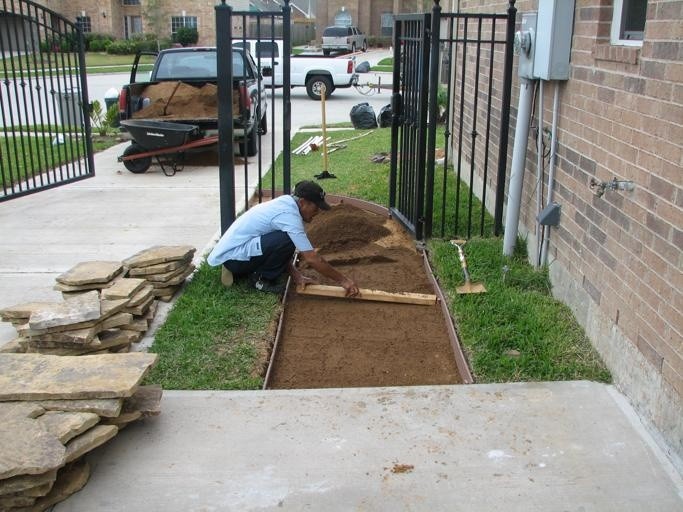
[55,86,84,127]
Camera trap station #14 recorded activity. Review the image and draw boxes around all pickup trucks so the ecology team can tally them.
[114,44,267,158]
[230,40,357,102]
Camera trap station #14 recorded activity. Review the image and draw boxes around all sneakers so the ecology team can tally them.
[221,264,234,287]
[251,273,280,294]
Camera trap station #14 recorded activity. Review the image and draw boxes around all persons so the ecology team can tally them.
[206,180,361,298]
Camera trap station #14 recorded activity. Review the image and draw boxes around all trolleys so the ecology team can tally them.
[116,118,217,178]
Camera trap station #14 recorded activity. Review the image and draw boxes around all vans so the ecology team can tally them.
[319,25,368,57]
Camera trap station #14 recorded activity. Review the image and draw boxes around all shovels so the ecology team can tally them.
[450,239,487,294]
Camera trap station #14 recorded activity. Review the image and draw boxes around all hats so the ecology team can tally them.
[294,180,331,210]
